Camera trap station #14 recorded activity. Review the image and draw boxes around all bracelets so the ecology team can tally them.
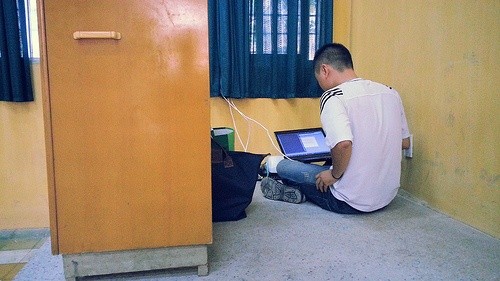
[331,168,344,180]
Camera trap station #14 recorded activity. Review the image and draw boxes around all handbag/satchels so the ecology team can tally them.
[211,136,272,223]
[211,127,235,152]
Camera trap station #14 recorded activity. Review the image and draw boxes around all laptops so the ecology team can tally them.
[274,128,332,162]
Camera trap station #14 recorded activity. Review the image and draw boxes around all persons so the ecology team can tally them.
[261,43,411,214]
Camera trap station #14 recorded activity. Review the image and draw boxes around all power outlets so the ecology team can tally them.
[403,133,413,159]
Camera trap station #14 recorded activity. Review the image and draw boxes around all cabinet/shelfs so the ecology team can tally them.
[35,0,213,281]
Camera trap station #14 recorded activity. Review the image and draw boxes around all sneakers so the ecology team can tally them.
[260,177,306,204]
